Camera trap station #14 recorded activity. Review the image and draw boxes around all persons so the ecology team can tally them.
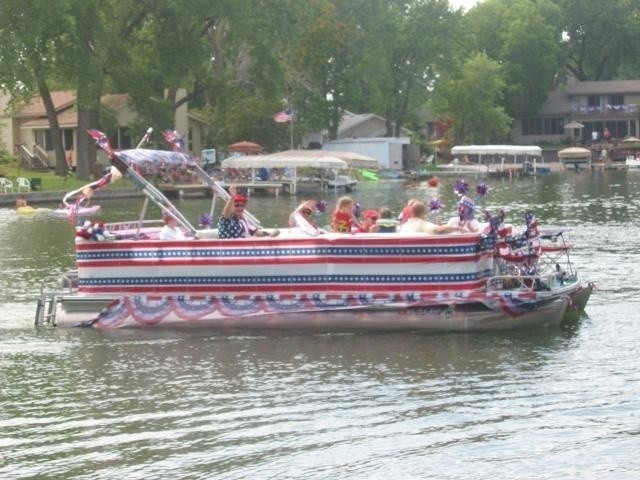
[399,198,417,224]
[330,196,354,234]
[592,129,599,142]
[447,196,481,234]
[16,193,27,208]
[378,208,398,233]
[398,201,467,234]
[159,204,193,239]
[216,185,273,239]
[603,127,610,142]
[358,209,378,233]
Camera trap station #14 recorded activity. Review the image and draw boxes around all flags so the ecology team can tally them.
[271,109,292,123]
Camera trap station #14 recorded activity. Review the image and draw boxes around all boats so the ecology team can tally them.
[47,130,614,332]
[557,141,605,177]
[445,142,553,176]
[269,148,380,192]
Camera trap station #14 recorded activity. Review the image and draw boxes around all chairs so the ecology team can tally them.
[0,177,30,194]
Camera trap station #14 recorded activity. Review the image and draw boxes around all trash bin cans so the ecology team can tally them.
[30,177,42,190]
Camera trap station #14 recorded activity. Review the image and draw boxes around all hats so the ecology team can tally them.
[457,196,475,210]
[234,194,248,202]
[365,210,378,218]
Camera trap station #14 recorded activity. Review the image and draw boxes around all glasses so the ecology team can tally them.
[234,204,245,208]
[302,211,311,215]
